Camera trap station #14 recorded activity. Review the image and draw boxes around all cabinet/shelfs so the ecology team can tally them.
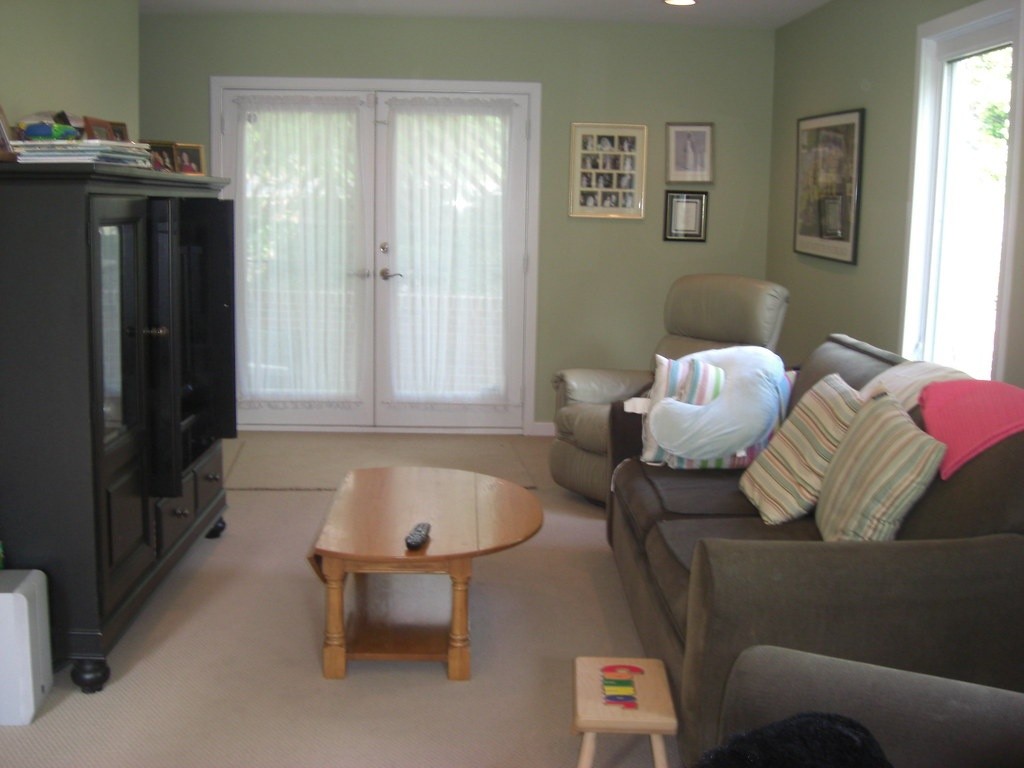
[0,162,237,696]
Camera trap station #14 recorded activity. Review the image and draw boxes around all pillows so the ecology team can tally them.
[737,373,868,525]
[816,381,947,541]
[639,353,690,466]
[665,358,798,469]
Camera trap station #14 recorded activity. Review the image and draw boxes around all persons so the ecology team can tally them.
[179,151,198,173]
[149,150,173,172]
[580,135,634,208]
[684,132,697,170]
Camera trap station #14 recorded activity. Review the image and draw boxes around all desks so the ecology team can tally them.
[307,466,543,682]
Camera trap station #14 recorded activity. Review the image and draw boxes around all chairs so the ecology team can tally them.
[695,646,1024,768]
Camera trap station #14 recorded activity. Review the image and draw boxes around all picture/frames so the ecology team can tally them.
[663,190,708,243]
[138,139,179,173]
[664,122,715,184]
[568,123,647,219]
[793,107,865,266]
[176,143,206,176]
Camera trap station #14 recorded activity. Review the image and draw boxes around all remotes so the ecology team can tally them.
[405,523,431,550]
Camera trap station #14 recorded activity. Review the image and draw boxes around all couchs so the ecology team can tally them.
[604,333,1024,768]
[549,273,793,504]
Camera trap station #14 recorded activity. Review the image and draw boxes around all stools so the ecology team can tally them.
[572,656,677,768]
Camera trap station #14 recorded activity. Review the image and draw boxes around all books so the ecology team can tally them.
[13,144,152,168]
